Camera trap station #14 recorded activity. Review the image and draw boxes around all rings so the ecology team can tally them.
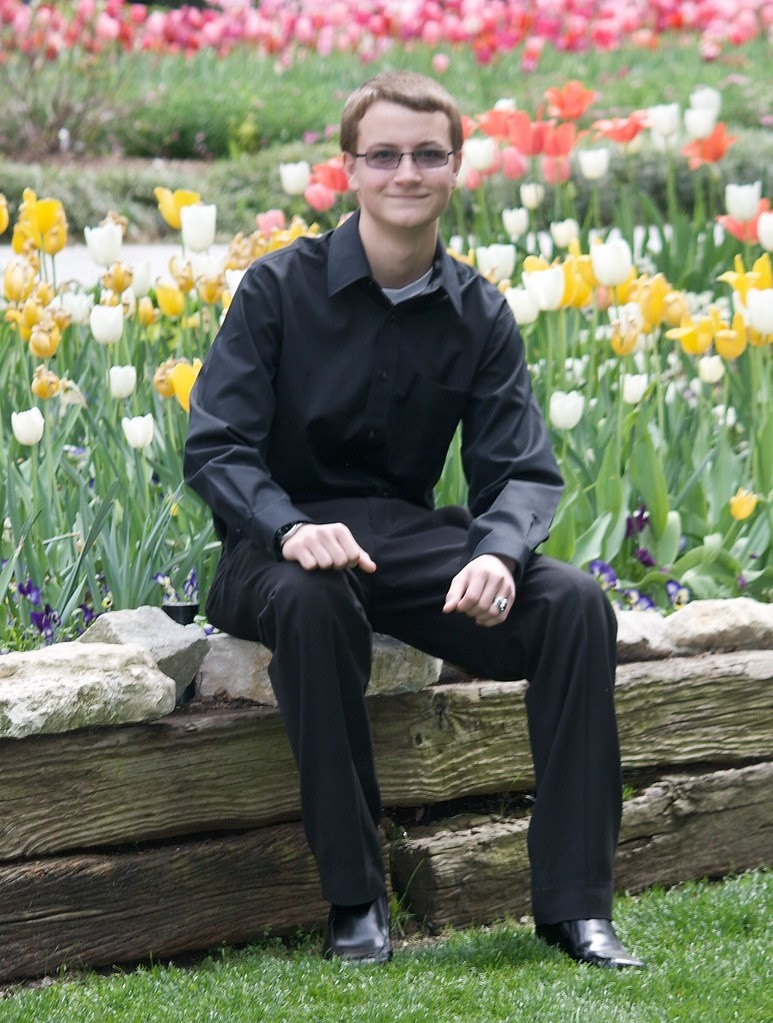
[497,598,508,612]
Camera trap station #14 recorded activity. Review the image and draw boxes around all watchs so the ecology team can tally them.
[276,521,306,547]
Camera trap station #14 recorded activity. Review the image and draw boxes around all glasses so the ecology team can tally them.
[355,150,456,169]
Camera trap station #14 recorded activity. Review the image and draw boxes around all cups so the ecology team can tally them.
[161,602,199,707]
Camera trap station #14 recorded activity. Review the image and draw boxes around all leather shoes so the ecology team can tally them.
[322,888,392,966]
[534,915,644,972]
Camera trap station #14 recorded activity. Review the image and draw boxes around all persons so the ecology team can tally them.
[183,69,647,972]
